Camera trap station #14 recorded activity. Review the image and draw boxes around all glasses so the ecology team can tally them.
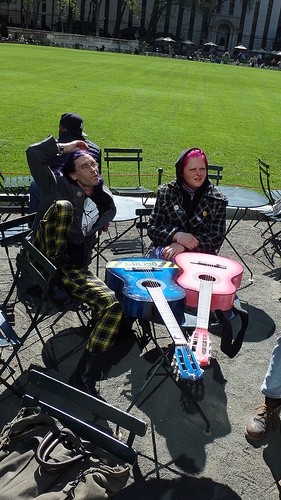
[76,163,98,172]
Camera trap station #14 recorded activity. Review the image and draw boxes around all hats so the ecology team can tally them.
[60,113,88,137]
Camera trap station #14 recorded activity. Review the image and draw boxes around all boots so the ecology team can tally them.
[49,272,71,305]
[70,349,106,404]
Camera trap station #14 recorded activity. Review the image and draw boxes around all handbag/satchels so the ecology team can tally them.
[0,406,130,500]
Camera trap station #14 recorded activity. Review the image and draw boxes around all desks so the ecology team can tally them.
[215,185,270,276]
[115,309,238,435]
[89,194,147,277]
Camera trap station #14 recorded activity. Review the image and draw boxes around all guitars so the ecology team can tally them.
[175,251,246,367]
[105,255,206,385]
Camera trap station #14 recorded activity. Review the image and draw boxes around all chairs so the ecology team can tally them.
[0,148,281,466]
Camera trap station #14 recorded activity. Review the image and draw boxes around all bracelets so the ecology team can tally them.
[58,144,64,156]
[177,232,183,244]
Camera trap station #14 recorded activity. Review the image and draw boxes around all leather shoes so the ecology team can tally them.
[244,397,281,443]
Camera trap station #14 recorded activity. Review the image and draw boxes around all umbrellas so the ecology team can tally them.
[250,48,281,56]
[155,36,176,43]
[204,41,218,46]
[182,40,195,46]
[235,45,247,49]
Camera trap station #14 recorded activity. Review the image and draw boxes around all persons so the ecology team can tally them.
[245,337,281,440]
[134,48,139,55]
[187,51,281,69]
[19,135,124,401]
[96,45,105,51]
[168,45,174,58]
[118,147,228,334]
[0,34,33,44]
[25,113,103,228]
